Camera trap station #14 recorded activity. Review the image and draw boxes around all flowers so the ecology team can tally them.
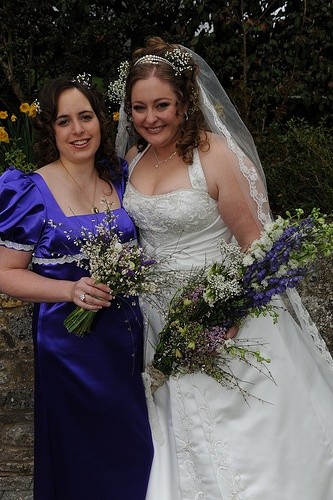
[40,197,192,339]
[141,208,333,408]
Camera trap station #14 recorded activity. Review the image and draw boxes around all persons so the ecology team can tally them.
[89,35,333,500]
[0,73,155,500]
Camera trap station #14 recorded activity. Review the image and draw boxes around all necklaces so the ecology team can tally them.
[153,147,177,168]
[60,159,99,214]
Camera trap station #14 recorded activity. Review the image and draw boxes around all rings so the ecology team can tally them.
[80,293,86,302]
